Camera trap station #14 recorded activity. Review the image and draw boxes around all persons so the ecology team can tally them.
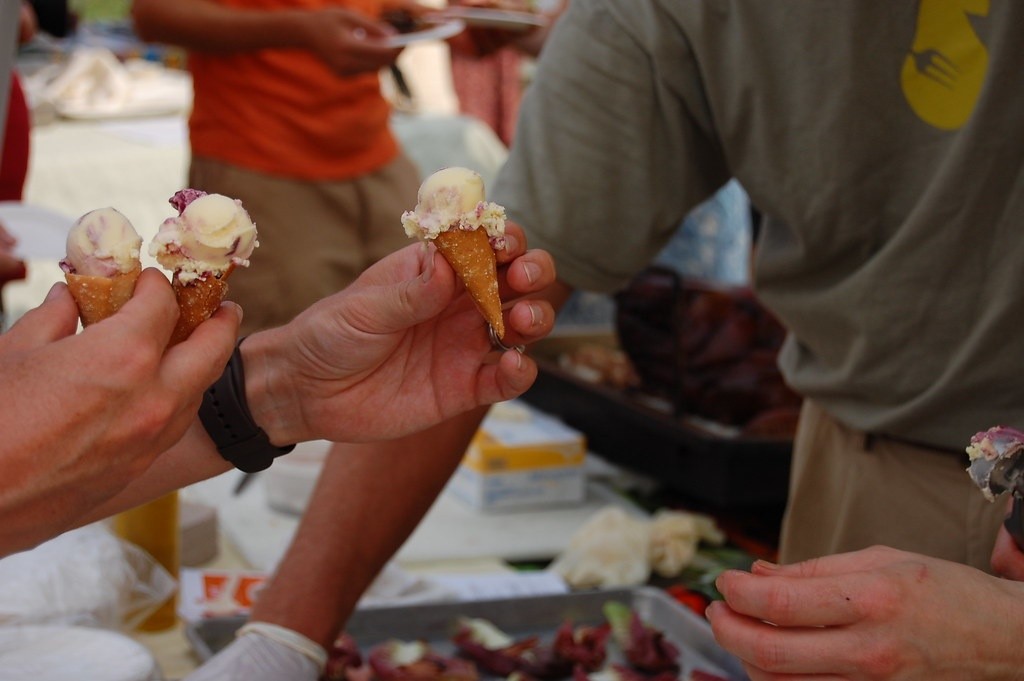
[0,0,80,204]
[965,426,1024,580]
[180,0,1024,681]
[0,221,556,559]
[131,0,542,326]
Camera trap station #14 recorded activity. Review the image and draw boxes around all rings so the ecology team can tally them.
[489,322,526,353]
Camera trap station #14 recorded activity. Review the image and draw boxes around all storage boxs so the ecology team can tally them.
[453,394,585,512]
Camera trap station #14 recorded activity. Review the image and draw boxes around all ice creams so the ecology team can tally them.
[152,189,257,342]
[59,206,144,328]
[401,168,508,340]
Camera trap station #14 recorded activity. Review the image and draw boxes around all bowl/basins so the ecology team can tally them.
[0,625,156,681]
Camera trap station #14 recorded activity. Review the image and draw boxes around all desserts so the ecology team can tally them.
[965,426,1024,552]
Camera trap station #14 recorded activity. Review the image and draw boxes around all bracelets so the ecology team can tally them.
[198,341,296,474]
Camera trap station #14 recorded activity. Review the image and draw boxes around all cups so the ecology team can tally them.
[111,490,181,633]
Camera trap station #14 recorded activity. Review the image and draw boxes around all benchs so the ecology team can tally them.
[534,356,790,535]
